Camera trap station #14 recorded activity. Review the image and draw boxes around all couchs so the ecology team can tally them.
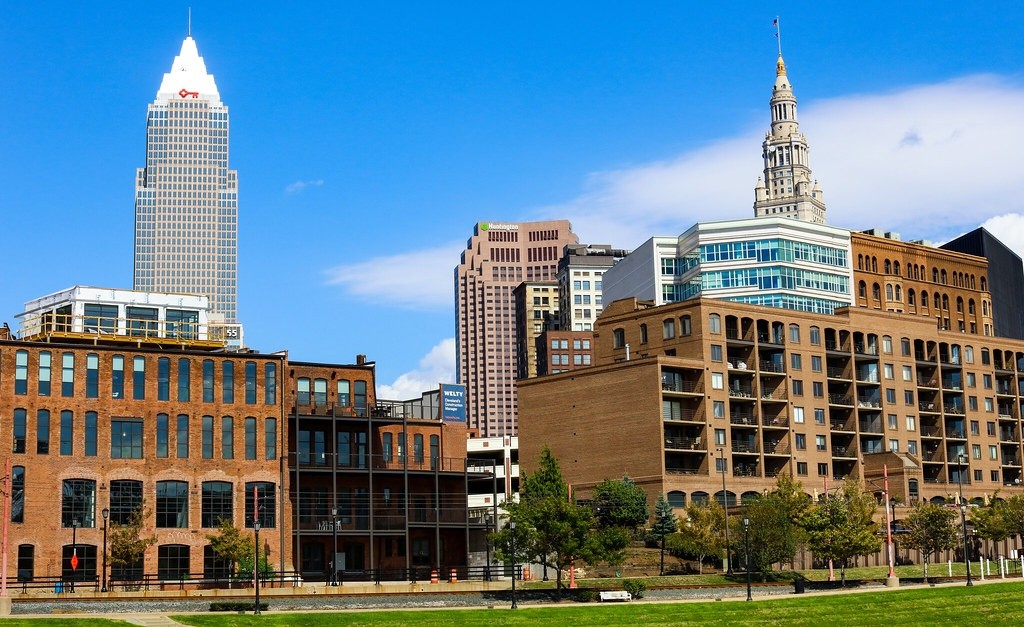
[678,440,691,450]
[664,438,676,449]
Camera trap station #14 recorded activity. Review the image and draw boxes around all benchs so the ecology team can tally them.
[599,590,632,602]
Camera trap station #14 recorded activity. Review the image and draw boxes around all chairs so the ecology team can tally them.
[318,520,342,530]
[694,436,702,450]
[371,406,392,417]
[770,418,780,426]
[742,417,751,425]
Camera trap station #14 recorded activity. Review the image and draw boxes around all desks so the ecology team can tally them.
[375,406,386,417]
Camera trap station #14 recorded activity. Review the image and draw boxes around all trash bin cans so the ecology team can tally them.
[794,577,806,592]
[54,583,63,592]
